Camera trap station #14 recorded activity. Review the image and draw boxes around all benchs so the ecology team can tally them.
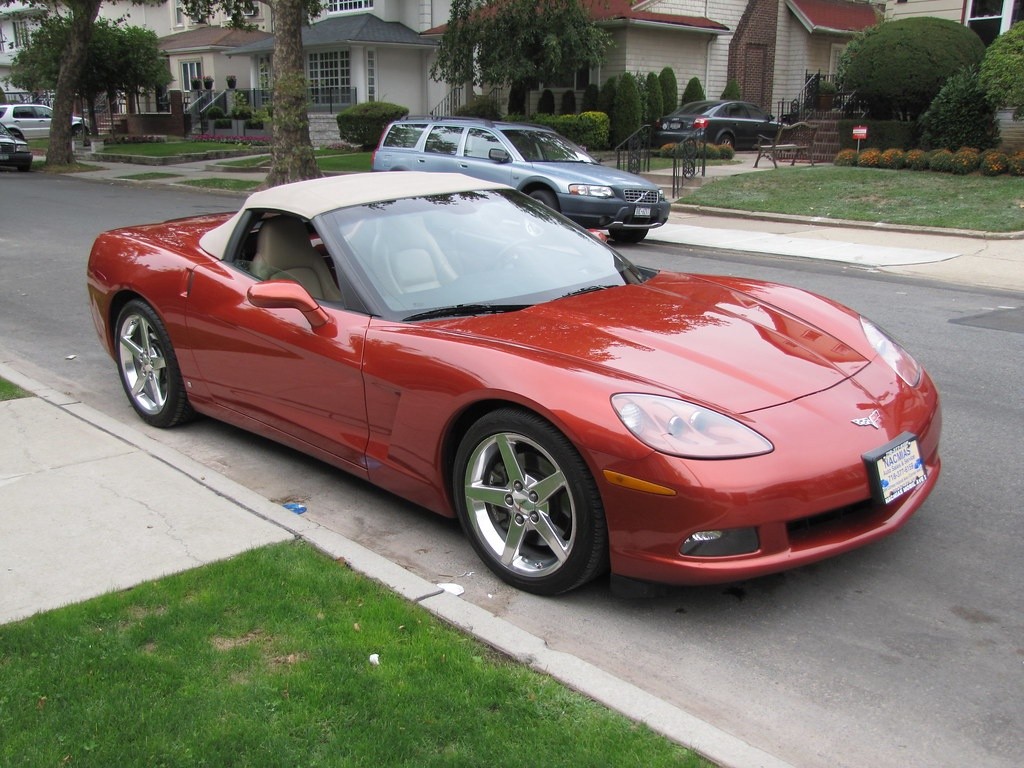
[754,121,819,169]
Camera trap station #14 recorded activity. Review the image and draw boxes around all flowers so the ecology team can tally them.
[191,76,202,85]
[203,76,214,83]
[226,75,236,81]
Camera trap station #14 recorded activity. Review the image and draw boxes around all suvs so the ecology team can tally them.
[371,114,671,244]
[0,104,90,141]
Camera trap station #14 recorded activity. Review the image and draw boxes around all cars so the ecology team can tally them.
[0,121,34,173]
[653,101,791,152]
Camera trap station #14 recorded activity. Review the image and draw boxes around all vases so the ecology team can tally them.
[204,81,213,90]
[192,82,202,90]
[227,80,236,89]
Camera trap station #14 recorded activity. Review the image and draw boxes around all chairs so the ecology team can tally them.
[250,215,343,302]
[370,213,459,296]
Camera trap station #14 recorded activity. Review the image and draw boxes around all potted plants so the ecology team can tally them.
[252,110,273,135]
[213,119,233,136]
[230,105,252,136]
[206,106,226,135]
[244,119,265,136]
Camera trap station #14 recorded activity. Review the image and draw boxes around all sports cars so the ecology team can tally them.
[86,170,944,598]
